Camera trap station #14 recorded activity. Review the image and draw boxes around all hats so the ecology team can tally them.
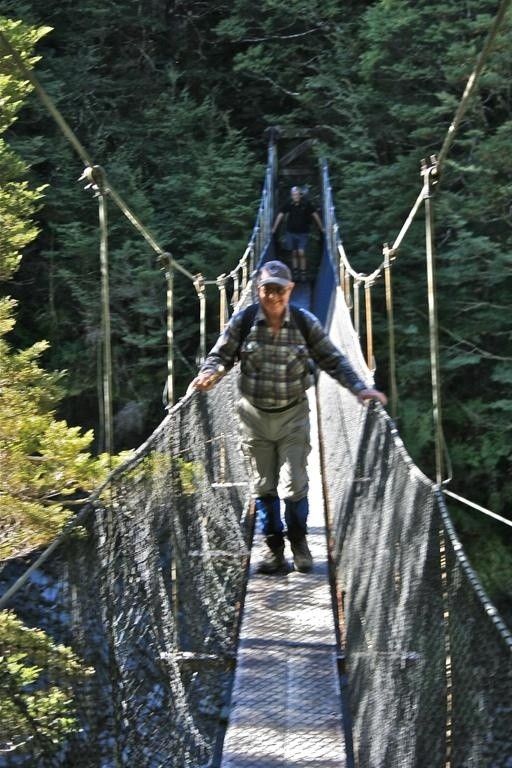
[257,261,292,288]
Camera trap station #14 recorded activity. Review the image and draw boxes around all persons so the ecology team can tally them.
[271,185,326,286]
[188,258,391,575]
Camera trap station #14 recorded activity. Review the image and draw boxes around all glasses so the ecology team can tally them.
[264,287,286,294]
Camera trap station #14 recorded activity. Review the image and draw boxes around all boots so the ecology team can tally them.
[289,535,312,572]
[256,542,284,573]
